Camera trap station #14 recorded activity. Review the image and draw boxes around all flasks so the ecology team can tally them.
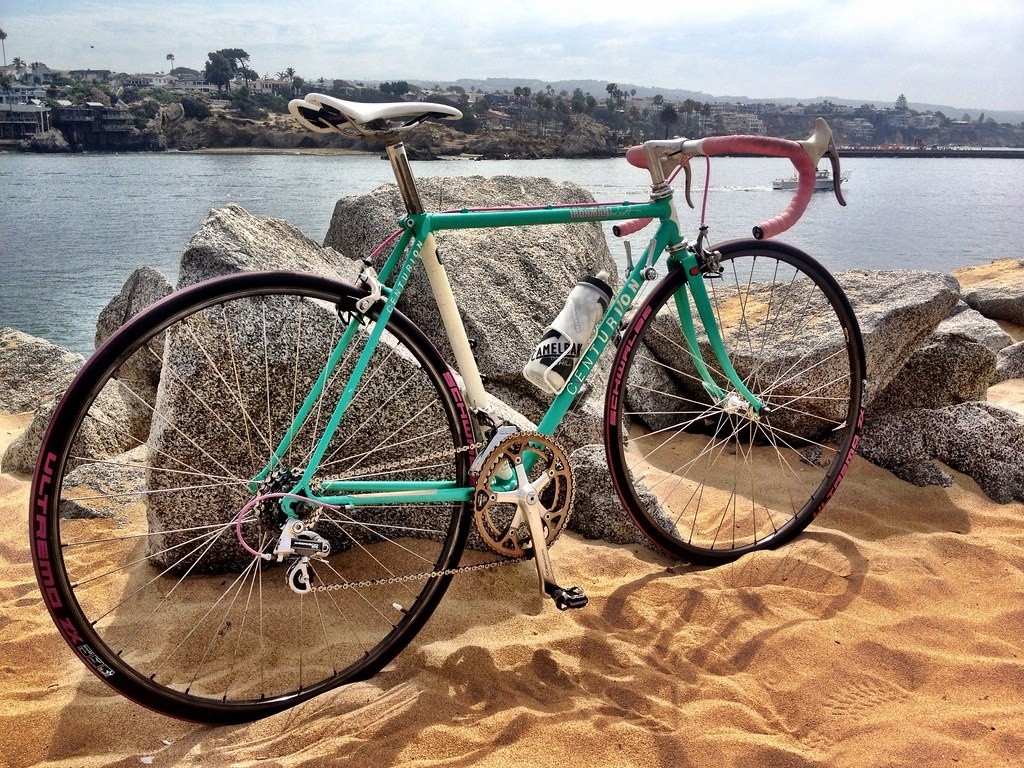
[523,269,615,392]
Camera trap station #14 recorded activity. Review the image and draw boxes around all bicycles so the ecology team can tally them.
[25,92,865,725]
